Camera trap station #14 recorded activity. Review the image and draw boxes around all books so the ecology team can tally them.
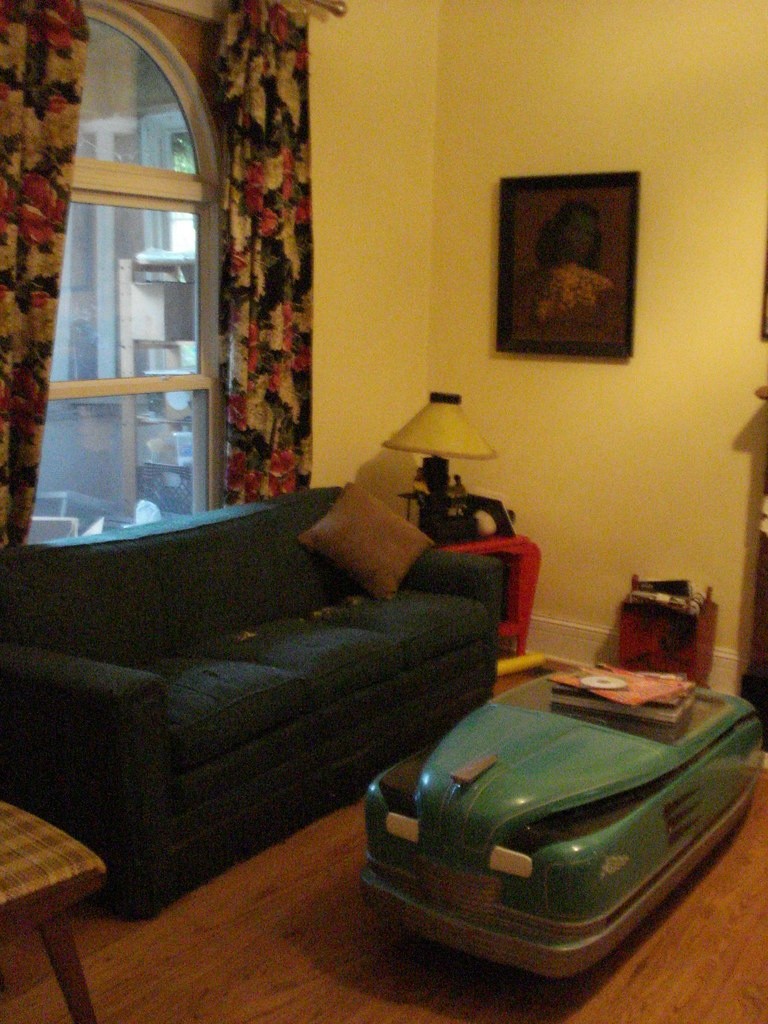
[545,660,697,726]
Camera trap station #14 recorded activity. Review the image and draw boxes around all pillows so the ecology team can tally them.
[299,482,434,601]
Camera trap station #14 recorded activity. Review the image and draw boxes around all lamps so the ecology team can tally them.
[381,392,496,543]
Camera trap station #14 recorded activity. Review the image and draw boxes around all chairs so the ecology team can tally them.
[0,794,105,1024]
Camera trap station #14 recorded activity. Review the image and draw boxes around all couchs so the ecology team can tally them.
[0,487,503,920]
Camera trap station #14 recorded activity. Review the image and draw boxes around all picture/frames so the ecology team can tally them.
[495,170,640,358]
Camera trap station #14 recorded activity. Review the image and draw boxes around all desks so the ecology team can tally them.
[437,530,541,657]
